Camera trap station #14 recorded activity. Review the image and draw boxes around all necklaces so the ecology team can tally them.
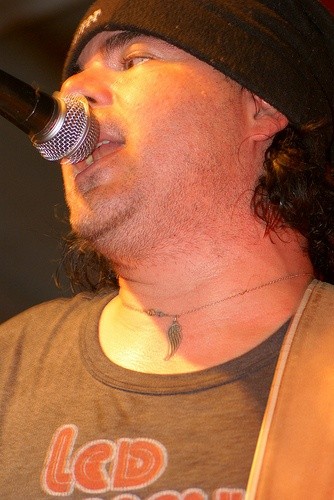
[117,272,315,361]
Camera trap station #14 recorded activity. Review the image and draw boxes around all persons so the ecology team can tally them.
[0,0,334,500]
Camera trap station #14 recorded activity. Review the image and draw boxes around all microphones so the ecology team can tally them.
[0,69,100,165]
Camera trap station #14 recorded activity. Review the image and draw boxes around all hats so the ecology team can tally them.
[61,0,334,132]
